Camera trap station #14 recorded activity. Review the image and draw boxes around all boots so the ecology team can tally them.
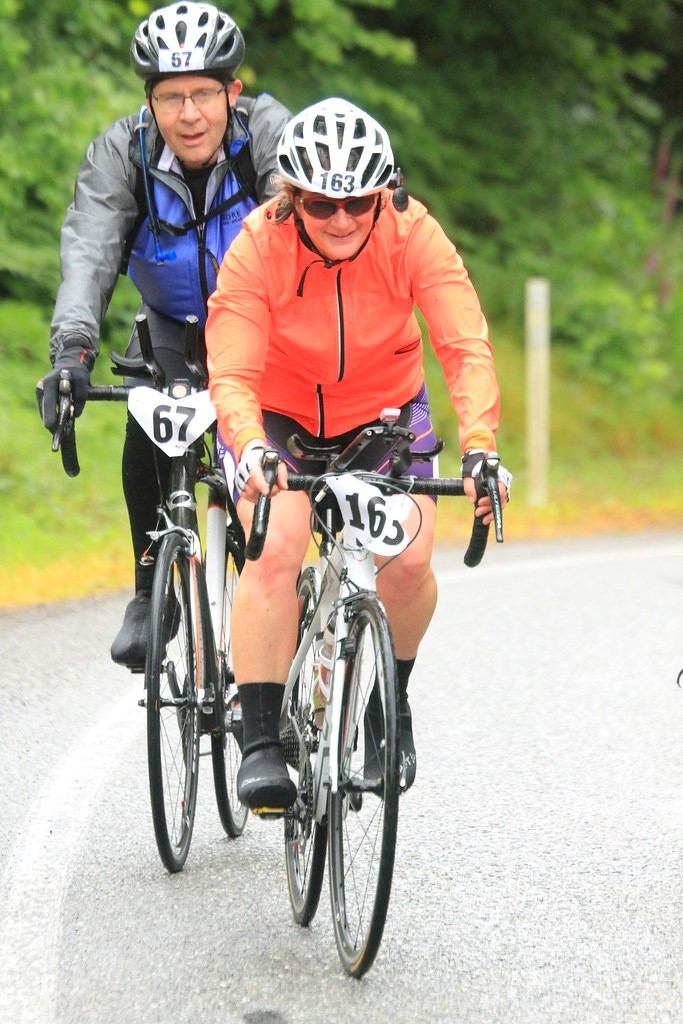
[363,656,416,800]
[110,561,181,666]
[237,682,298,808]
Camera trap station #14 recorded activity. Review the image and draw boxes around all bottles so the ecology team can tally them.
[313,632,325,729]
[318,616,337,705]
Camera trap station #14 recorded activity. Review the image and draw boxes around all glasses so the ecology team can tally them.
[295,194,377,220]
[152,86,226,113]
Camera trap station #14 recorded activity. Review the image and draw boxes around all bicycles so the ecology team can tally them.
[50,313,252,874]
[243,408,503,978]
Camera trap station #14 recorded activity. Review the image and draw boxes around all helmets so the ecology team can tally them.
[129,1,245,81]
[276,97,394,198]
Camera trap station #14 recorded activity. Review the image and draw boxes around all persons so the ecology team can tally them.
[37,0,296,667]
[205,98,512,808]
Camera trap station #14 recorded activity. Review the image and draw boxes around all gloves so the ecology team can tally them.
[36,346,96,435]
